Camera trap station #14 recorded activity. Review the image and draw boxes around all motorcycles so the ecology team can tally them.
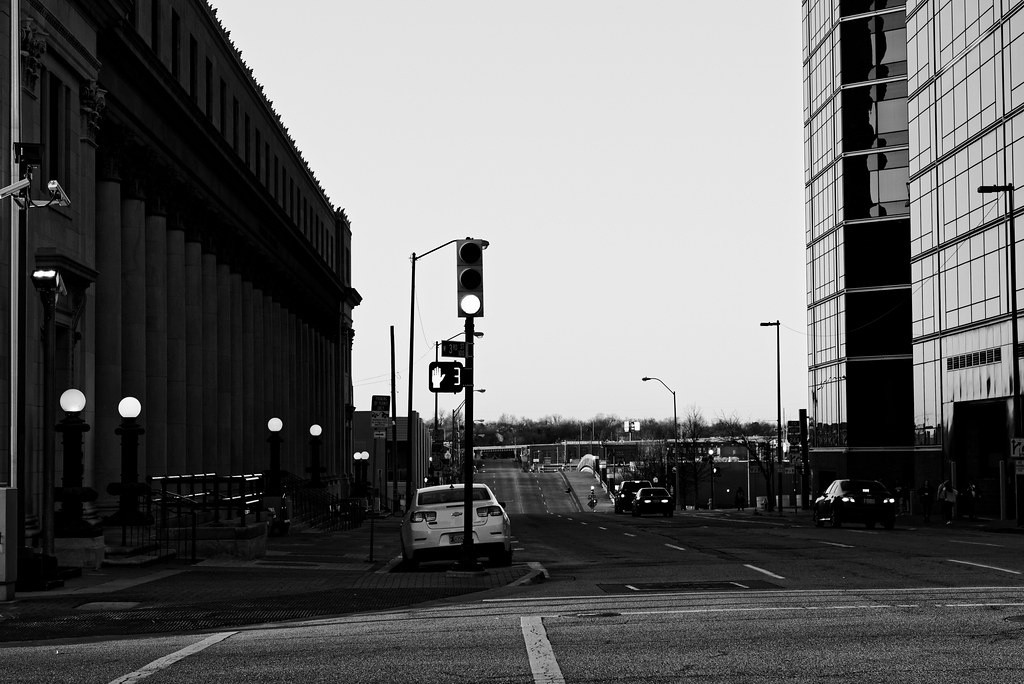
[588,497,598,509]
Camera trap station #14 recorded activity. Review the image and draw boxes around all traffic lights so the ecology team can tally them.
[712,467,719,476]
[456,240,485,316]
[429,362,463,394]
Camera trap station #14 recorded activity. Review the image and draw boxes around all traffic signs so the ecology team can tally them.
[1010,438,1024,457]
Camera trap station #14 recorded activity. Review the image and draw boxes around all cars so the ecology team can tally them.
[631,487,674,518]
[814,479,897,529]
[394,482,513,569]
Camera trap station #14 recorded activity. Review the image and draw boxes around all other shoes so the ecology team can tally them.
[923,516,930,523]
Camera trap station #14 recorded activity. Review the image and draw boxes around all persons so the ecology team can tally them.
[919,480,933,523]
[588,490,598,503]
[938,480,958,525]
[736,487,746,511]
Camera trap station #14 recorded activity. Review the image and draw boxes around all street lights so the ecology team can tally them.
[977,184,1022,520]
[406,239,489,512]
[642,377,679,511]
[434,332,484,432]
[452,389,486,466]
[760,321,782,515]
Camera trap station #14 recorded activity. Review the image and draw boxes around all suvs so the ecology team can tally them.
[615,480,652,514]
